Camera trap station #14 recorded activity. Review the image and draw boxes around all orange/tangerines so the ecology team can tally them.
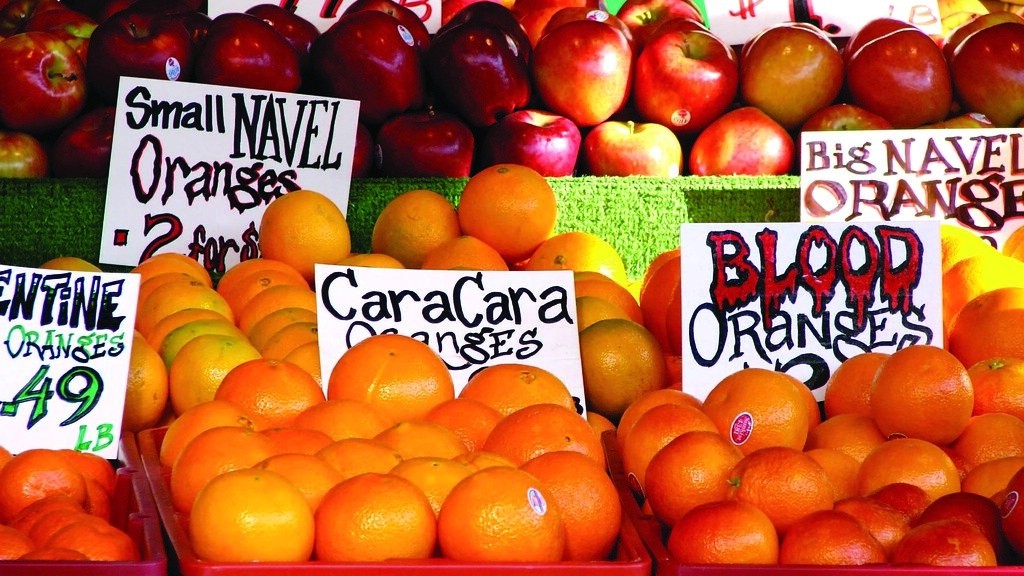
[0,443,145,562]
[31,161,1024,569]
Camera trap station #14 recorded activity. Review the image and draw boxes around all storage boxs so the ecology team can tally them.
[0,418,167,576]
[138,413,653,576]
[603,418,1024,576]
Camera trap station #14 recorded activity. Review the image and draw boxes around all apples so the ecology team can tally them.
[0,0,1024,180]
